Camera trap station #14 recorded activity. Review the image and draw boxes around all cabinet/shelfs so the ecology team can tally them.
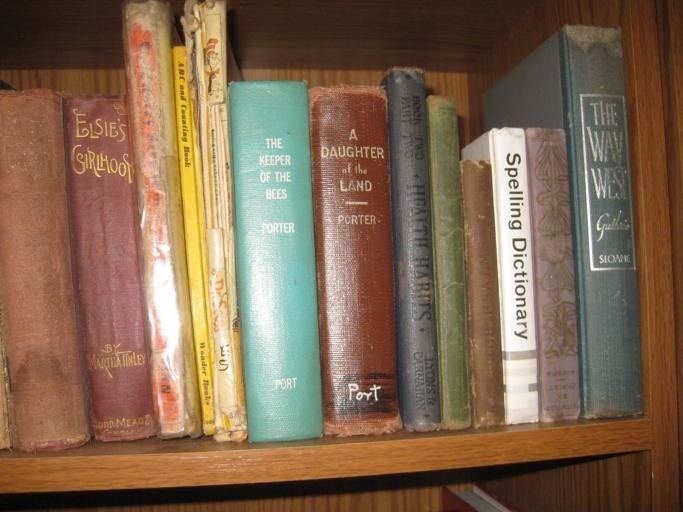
[1,0,681,512]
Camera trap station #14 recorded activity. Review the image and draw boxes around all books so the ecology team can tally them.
[174,46,221,440]
[525,125,582,424]
[427,95,472,431]
[306,80,401,437]
[64,90,156,442]
[443,479,515,512]
[227,78,323,442]
[0,85,91,454]
[479,22,645,417]
[379,66,440,431]
[457,124,539,427]
[459,156,506,429]
[119,0,202,442]
[188,0,249,442]
